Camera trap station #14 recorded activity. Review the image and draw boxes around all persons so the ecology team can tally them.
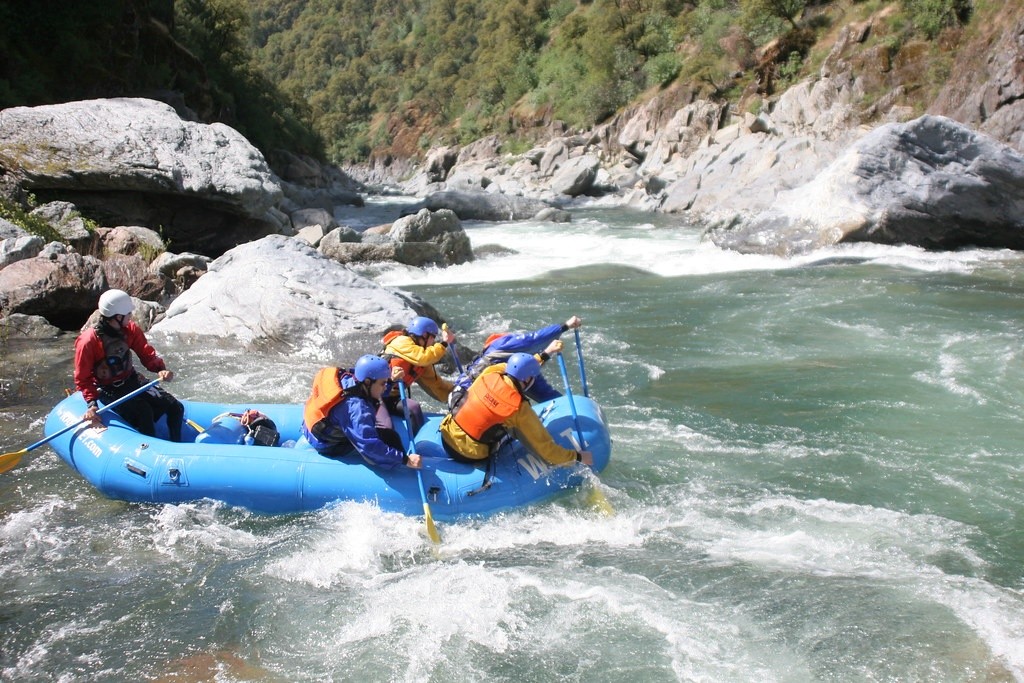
[378,315,592,467]
[298,353,423,470]
[74,289,185,444]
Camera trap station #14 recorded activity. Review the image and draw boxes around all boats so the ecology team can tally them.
[43,389,613,523]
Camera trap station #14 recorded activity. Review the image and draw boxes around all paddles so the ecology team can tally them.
[557,342,612,519]
[1,377,165,474]
[572,323,588,398]
[394,368,439,544]
[181,415,205,434]
[442,323,464,375]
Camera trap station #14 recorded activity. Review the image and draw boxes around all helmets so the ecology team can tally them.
[99,288,136,318]
[407,318,440,338]
[355,353,392,382]
[506,352,543,381]
